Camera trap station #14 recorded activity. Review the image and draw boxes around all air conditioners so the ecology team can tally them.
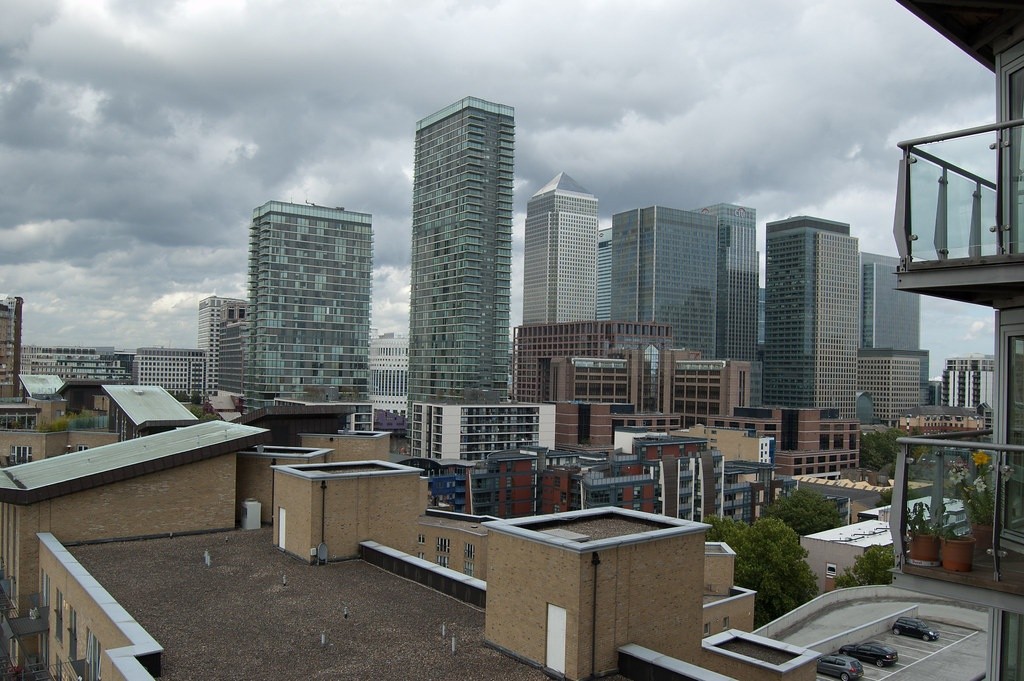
[236,501,262,530]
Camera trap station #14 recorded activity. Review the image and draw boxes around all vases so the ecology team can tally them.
[971,521,1002,550]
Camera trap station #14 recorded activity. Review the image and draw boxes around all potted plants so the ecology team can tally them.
[903,502,976,572]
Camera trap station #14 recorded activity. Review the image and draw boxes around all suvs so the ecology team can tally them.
[892,616,940,642]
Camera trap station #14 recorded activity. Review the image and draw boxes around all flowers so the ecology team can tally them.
[946,450,1006,526]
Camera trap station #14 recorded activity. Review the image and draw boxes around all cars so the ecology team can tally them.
[817,653,865,681]
[838,640,899,668]
